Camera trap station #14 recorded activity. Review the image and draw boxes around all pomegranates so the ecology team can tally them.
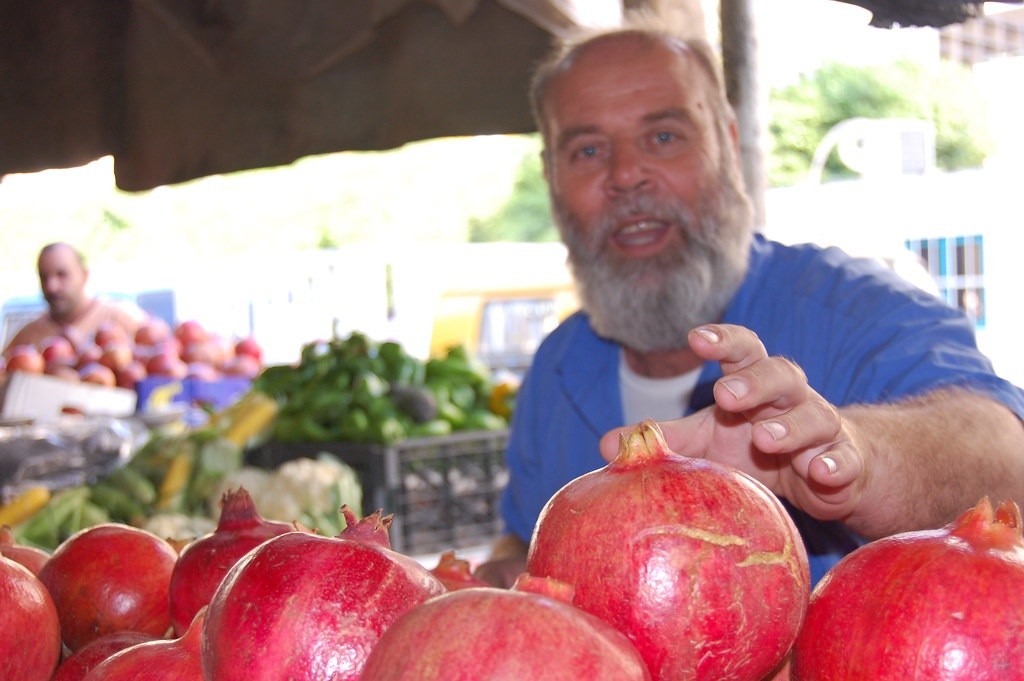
[0,417,1024,681]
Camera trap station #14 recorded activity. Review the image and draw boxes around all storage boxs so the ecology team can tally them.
[245,429,516,557]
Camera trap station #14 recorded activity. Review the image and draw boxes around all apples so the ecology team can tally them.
[0,319,262,394]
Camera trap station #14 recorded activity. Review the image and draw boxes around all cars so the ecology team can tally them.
[425,282,586,429]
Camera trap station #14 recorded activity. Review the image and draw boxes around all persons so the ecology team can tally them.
[2,243,147,355]
[473,30,1024,592]
